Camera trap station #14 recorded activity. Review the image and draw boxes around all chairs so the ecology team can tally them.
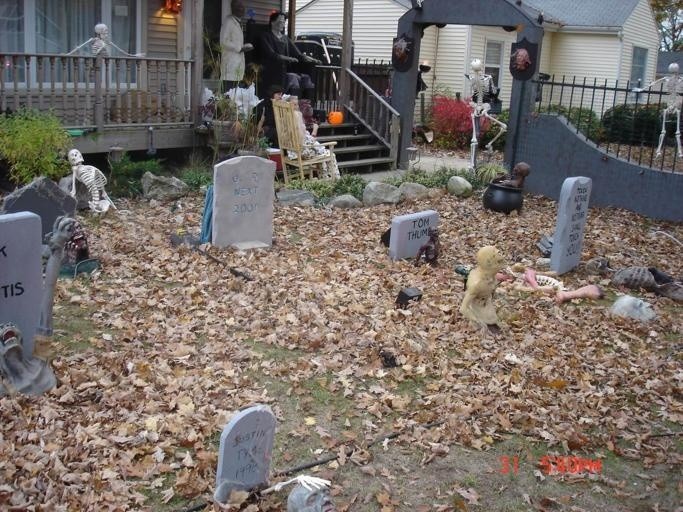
[272,99,338,185]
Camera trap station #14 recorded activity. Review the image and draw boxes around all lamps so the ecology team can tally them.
[164,0,183,15]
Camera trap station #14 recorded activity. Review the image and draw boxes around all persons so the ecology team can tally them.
[501,160,532,187]
[459,245,526,333]
[257,12,322,107]
[286,96,341,180]
[253,84,287,148]
[218,0,255,98]
[415,226,443,267]
[0,215,74,396]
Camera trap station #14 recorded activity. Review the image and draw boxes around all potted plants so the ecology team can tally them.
[202,32,270,157]
